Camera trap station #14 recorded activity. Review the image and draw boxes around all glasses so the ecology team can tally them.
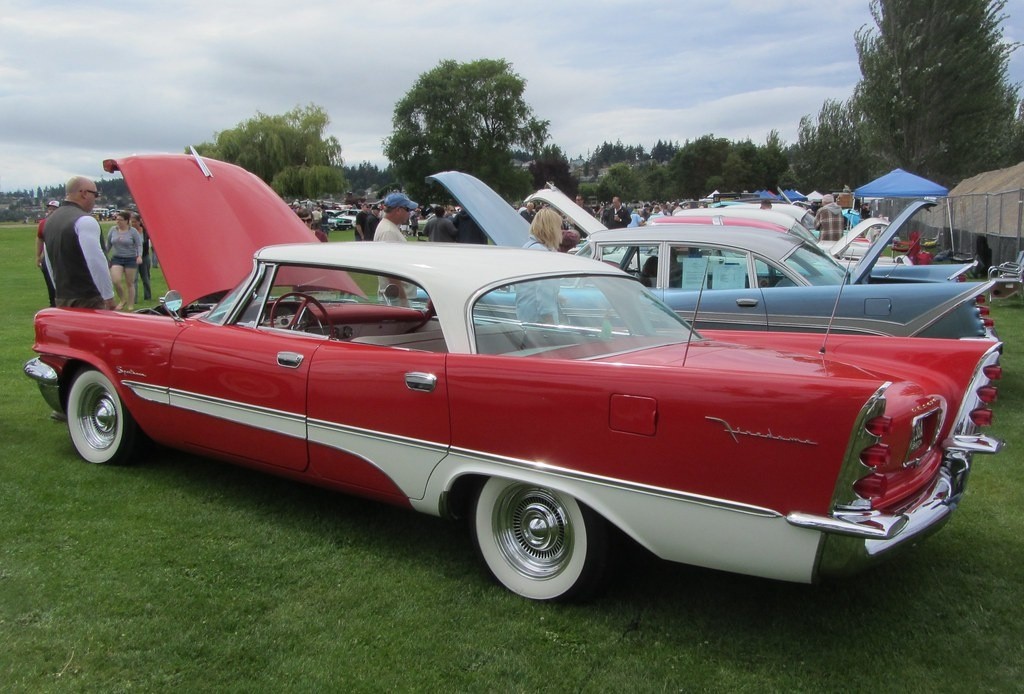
[79,189,98,198]
[400,207,410,212]
[300,218,313,224]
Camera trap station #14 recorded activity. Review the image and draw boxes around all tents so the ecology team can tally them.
[854,168,954,259]
[707,188,824,204]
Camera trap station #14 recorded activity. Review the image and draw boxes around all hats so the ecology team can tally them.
[383,193,419,210]
[46,200,60,208]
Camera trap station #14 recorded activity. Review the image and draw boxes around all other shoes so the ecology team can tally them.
[129,305,134,310]
[119,301,127,307]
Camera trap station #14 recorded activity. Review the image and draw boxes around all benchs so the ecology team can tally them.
[350,322,539,351]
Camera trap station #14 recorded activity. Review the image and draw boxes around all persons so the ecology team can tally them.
[517,194,683,252]
[861,203,871,219]
[409,203,488,245]
[105,212,143,310]
[813,194,844,241]
[288,201,381,241]
[373,193,418,307]
[131,213,152,304]
[36,200,61,307]
[516,207,563,326]
[43,175,117,421]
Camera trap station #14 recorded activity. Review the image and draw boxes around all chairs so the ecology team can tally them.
[951,251,975,278]
[892,241,910,256]
[988,250,1024,303]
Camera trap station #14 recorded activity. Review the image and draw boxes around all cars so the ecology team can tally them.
[646,201,912,268]
[415,169,987,369]
[25,154,998,600]
[325,209,362,230]
[522,187,978,283]
